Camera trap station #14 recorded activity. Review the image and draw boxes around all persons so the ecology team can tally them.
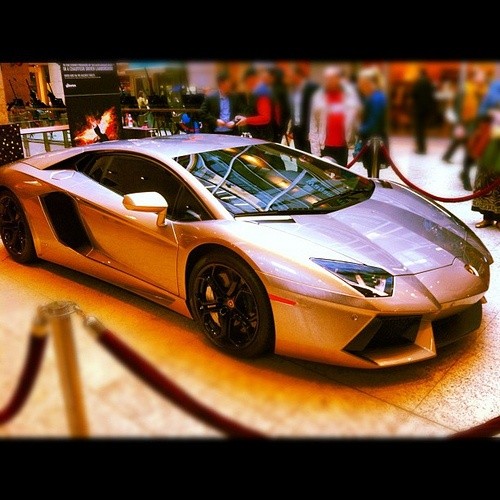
[470,102,500,230]
[401,65,438,155]
[193,69,245,137]
[281,63,321,153]
[441,67,500,191]
[263,64,292,145]
[352,66,391,187]
[137,90,151,110]
[233,67,281,148]
[307,64,364,172]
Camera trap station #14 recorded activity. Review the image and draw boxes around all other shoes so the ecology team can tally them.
[475,218,494,228]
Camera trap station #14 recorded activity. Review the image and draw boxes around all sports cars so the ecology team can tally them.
[0,123,494,371]
